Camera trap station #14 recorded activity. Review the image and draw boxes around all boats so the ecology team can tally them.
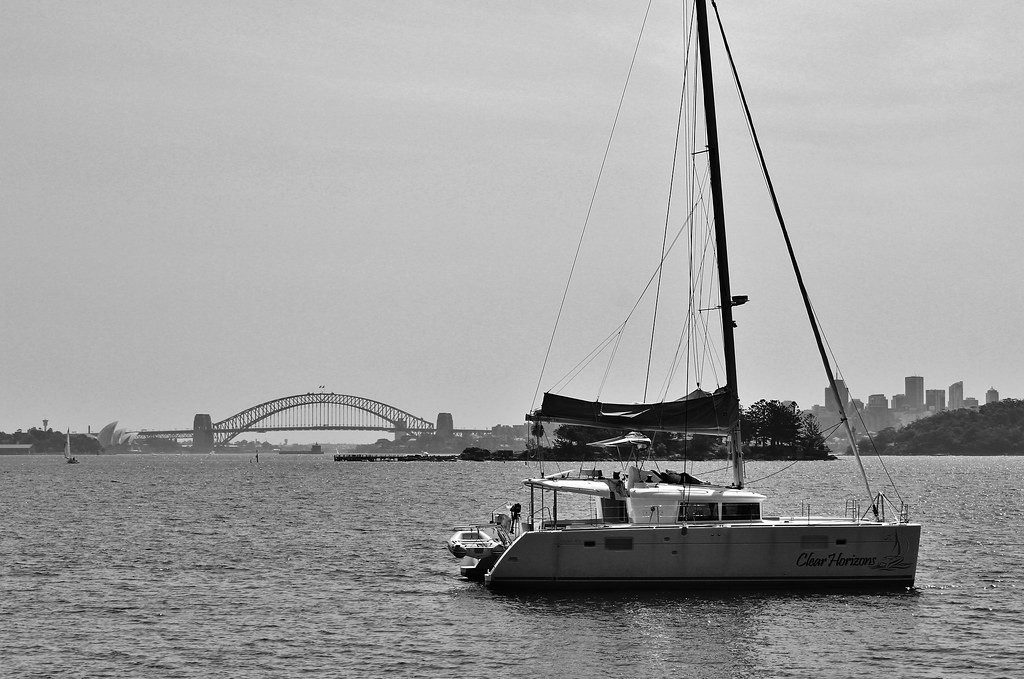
[448,531,504,558]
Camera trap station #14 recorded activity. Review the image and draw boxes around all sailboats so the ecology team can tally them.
[64,428,80,464]
[453,0,923,593]
